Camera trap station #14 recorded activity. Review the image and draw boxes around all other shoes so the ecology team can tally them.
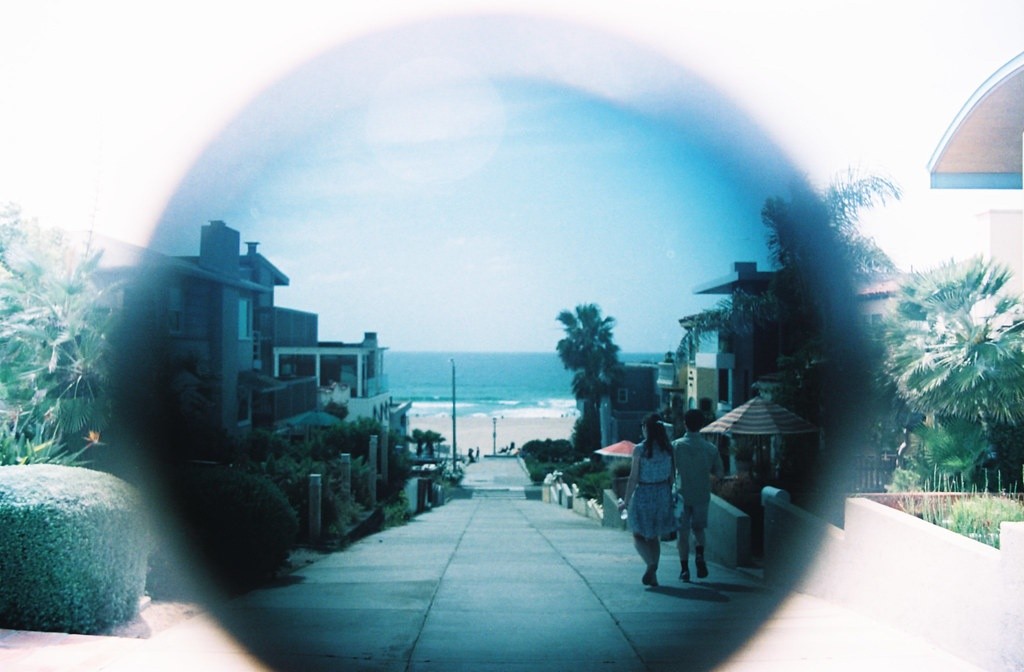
[680,570,690,582]
[642,563,658,587]
[696,557,708,578]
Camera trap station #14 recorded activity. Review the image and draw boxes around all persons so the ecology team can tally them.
[671,409,725,582]
[616,414,677,589]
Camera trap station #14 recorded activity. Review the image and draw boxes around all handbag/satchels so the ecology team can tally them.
[671,492,685,518]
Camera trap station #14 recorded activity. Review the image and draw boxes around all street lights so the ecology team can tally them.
[492,414,506,457]
[447,357,459,475]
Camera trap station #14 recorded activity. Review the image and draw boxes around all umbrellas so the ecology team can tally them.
[700,395,823,491]
[594,440,637,457]
